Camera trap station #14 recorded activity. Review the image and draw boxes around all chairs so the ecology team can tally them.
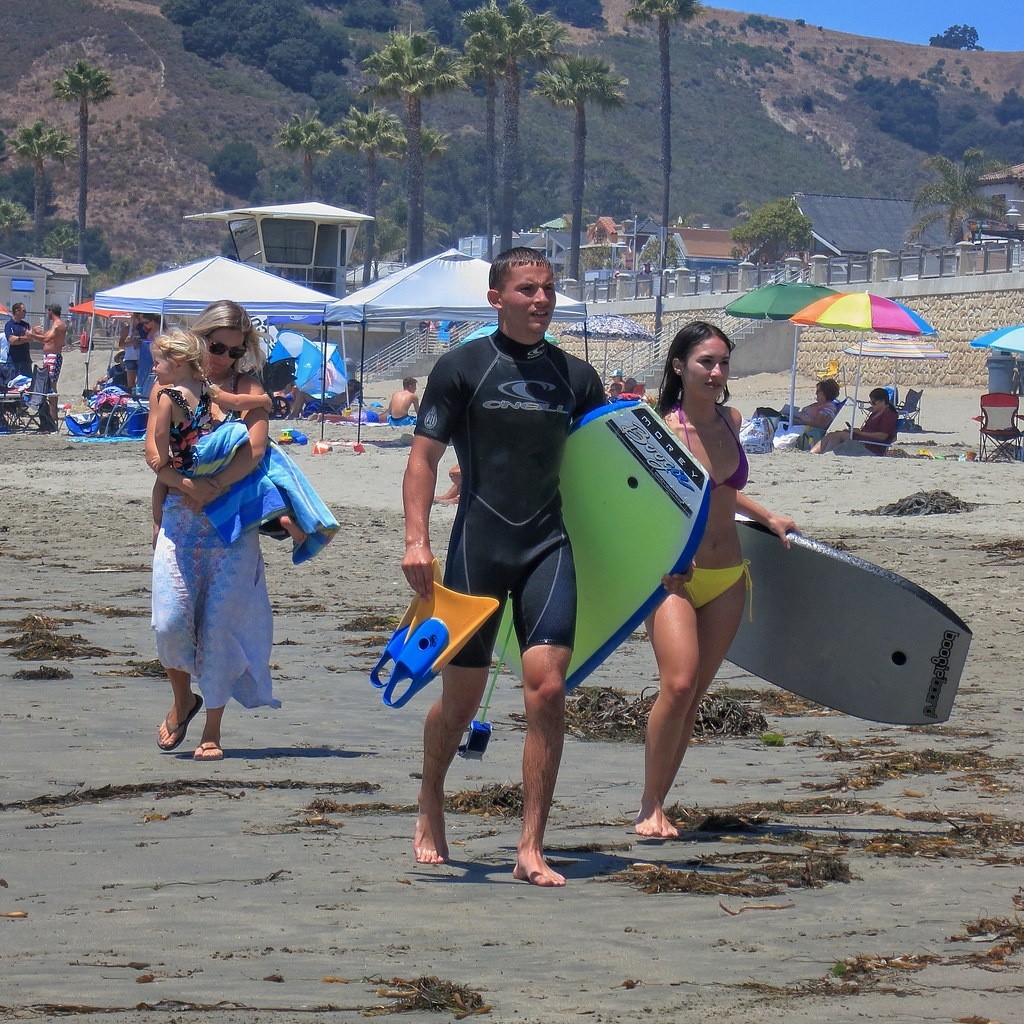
[801,397,849,451]
[859,420,904,457]
[0,362,158,438]
[315,387,364,414]
[857,384,900,429]
[972,393,1024,463]
[895,387,925,432]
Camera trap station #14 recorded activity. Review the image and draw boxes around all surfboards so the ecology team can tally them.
[495,401,709,692]
[723,519,973,727]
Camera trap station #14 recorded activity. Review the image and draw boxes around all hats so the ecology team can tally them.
[114,349,125,363]
[609,370,622,377]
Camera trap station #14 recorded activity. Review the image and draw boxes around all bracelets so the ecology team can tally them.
[19,336,20,341]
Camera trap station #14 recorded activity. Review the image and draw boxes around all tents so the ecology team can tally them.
[69,299,136,391]
[84,255,357,410]
[324,248,589,443]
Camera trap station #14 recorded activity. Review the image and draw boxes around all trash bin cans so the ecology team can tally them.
[1015,355,1024,395]
[346,358,357,380]
[987,347,1015,394]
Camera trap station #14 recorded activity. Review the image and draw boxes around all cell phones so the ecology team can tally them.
[845,422,851,428]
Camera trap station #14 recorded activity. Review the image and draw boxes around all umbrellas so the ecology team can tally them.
[559,313,654,386]
[844,334,948,392]
[725,280,842,429]
[970,325,1024,353]
[788,289,938,440]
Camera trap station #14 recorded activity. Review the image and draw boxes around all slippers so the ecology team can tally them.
[194,745,224,761]
[157,693,203,751]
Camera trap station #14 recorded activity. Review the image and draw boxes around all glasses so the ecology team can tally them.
[205,334,247,359]
[610,388,617,392]
[141,319,153,325]
[868,399,881,406]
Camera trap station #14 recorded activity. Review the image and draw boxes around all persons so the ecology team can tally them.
[288,378,362,421]
[433,461,462,506]
[144,299,308,761]
[419,321,468,351]
[778,378,840,431]
[387,376,420,426]
[632,321,801,836]
[118,312,167,392]
[811,387,898,457]
[401,248,605,885]
[5,303,42,377]
[604,369,636,404]
[25,304,65,426]
[710,440,723,448]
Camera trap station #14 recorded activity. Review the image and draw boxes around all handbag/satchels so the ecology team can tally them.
[7,375,32,388]
[800,430,821,450]
[65,413,100,437]
[739,418,773,454]
[772,421,805,450]
